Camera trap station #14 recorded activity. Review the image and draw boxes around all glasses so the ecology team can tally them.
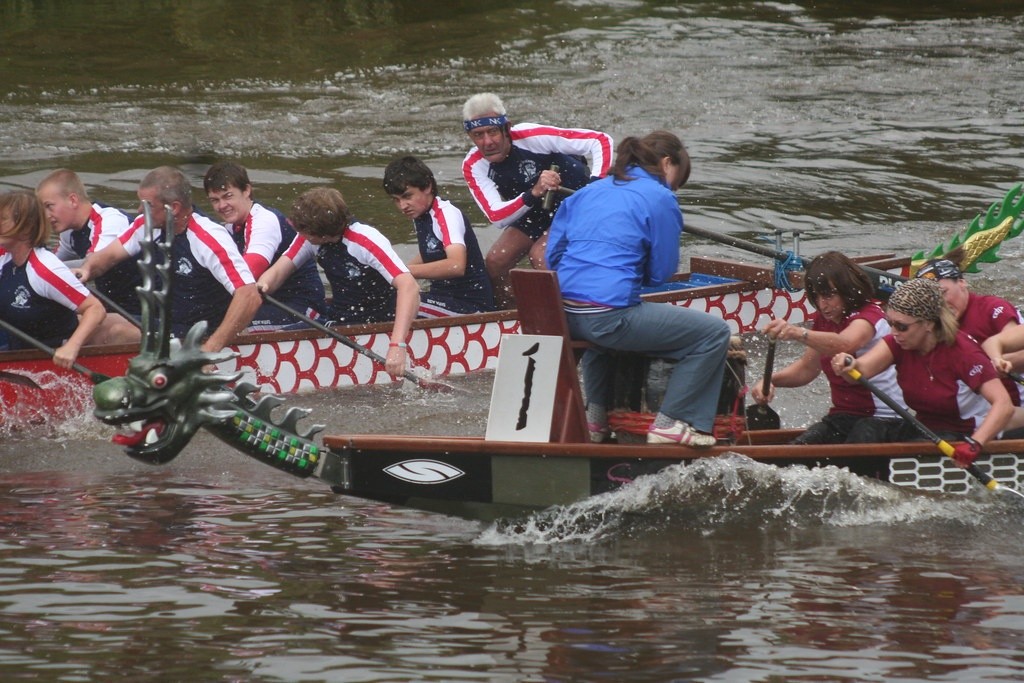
[886,320,925,332]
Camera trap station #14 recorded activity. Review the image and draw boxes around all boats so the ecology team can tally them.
[0,177,1024,432]
[90,200,1024,537]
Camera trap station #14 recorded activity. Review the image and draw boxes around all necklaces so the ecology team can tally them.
[918,350,935,381]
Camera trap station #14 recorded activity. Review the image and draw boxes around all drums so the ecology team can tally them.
[611,334,749,444]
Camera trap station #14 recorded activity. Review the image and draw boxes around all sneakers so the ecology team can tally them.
[647,420,717,445]
[588,423,611,442]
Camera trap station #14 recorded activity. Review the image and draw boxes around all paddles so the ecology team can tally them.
[539,163,910,304]
[257,283,467,395]
[1003,362,1024,386]
[0,317,112,385]
[843,355,1024,502]
[745,327,781,431]
[76,271,258,408]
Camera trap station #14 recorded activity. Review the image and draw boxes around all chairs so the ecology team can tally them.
[508,266,593,444]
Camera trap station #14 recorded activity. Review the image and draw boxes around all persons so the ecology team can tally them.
[0,189,107,370]
[383,157,497,319]
[831,278,1015,476]
[752,251,916,445]
[257,187,421,378]
[204,159,326,335]
[924,259,1024,440]
[70,166,262,372]
[545,131,730,446]
[37,169,142,346]
[462,92,613,311]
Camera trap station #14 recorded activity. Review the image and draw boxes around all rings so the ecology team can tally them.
[780,331,784,336]
[834,362,843,368]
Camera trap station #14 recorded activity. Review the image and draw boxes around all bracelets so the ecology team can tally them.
[799,326,808,343]
[389,342,407,348]
